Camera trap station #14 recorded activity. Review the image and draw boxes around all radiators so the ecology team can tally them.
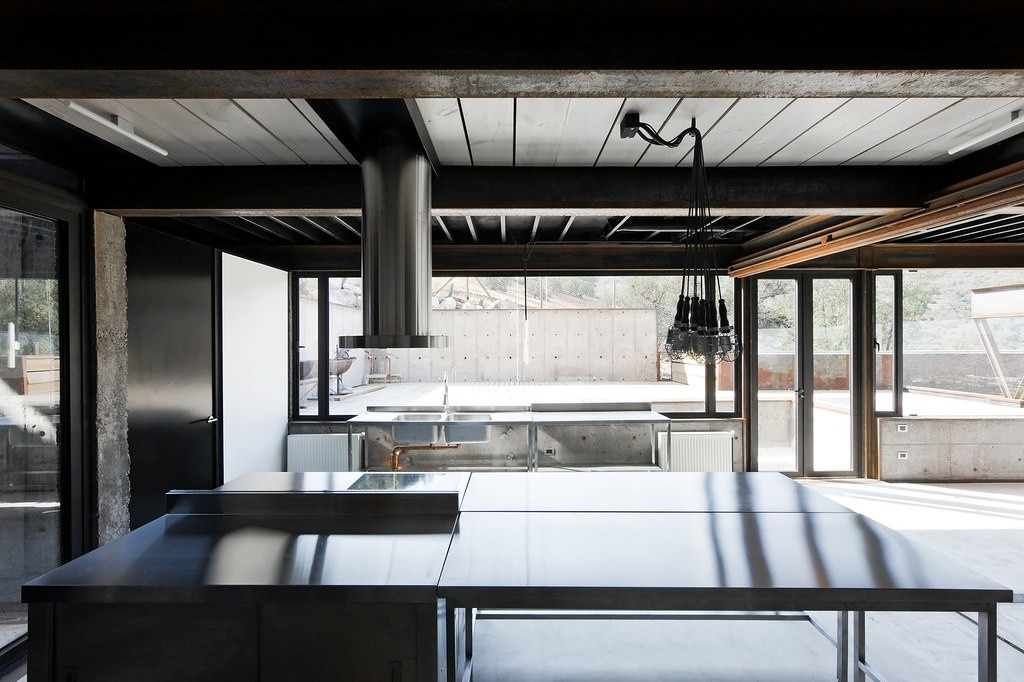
[286,430,366,471]
[658,430,738,472]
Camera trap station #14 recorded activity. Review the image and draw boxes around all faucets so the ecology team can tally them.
[443,372,450,406]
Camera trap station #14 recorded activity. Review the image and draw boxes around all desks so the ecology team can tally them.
[21,470,1015,682]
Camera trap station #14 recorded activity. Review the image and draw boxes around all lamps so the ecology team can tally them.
[621,115,742,365]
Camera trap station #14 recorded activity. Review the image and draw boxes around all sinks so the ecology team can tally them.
[390,413,442,443]
[445,414,492,443]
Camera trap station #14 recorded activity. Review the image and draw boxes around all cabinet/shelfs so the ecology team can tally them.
[878,418,1024,481]
